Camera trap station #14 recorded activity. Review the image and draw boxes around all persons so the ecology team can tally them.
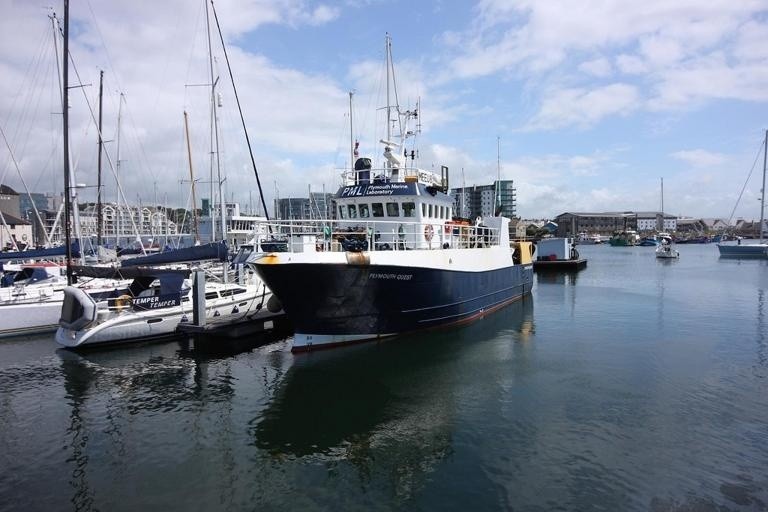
[397,223,408,251]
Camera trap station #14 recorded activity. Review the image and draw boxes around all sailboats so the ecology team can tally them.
[651,177,679,259]
[2,0,281,357]
[714,126,767,261]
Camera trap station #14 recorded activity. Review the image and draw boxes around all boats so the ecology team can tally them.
[588,227,742,249]
[245,31,536,349]
